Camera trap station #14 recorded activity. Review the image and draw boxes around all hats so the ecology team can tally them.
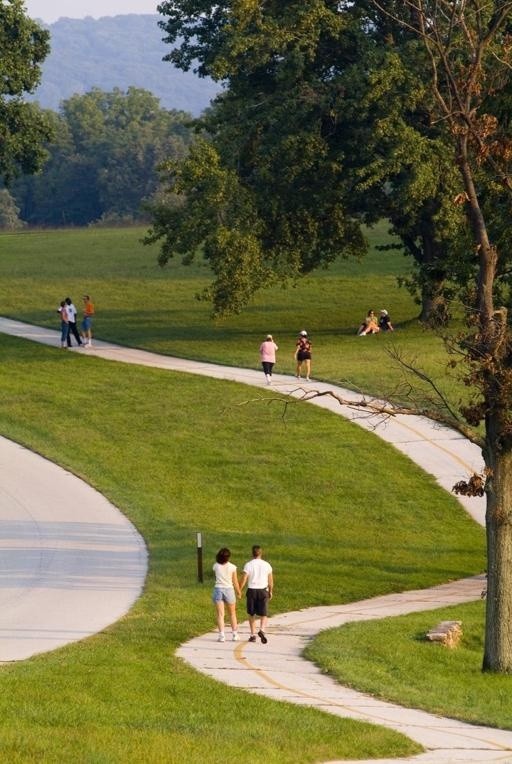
[267,335,272,340]
[380,309,388,316]
[299,330,307,336]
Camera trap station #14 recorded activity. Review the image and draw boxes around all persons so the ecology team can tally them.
[258,334,279,385]
[57,296,83,346]
[359,308,394,336]
[295,329,314,379]
[59,300,69,348]
[80,294,95,347]
[209,548,241,641]
[241,542,275,646]
[357,309,377,335]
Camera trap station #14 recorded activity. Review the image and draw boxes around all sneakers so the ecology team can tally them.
[360,332,366,336]
[249,636,256,642]
[258,630,267,644]
[232,635,237,641]
[218,636,225,642]
